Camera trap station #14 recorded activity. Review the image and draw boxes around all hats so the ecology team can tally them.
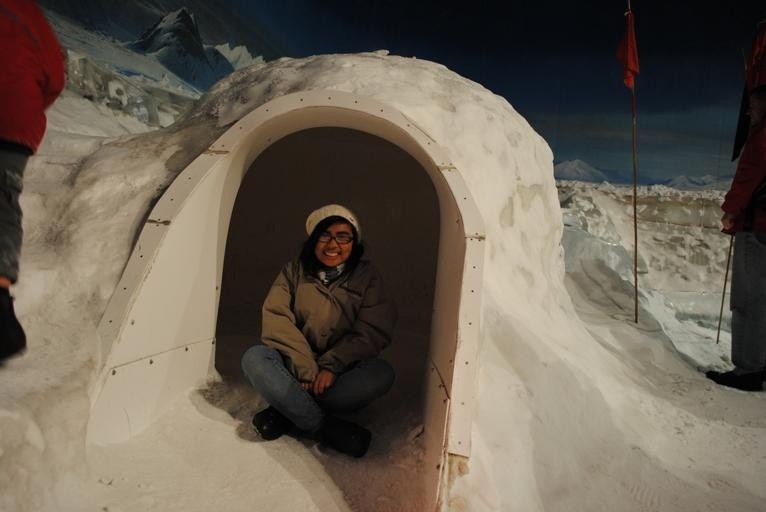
[306,204,362,244]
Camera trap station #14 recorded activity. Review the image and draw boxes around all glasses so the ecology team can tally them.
[319,232,353,244]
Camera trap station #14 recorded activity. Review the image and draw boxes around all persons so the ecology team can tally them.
[705,83,766,391]
[240,203,398,458]
[2,0,66,359]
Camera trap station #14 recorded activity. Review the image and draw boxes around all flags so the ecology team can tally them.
[731,21,766,162]
[614,9,640,97]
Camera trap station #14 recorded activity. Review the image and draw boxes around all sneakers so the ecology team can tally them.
[309,415,372,459]
[0,286,26,361]
[251,405,284,441]
[706,368,764,390]
